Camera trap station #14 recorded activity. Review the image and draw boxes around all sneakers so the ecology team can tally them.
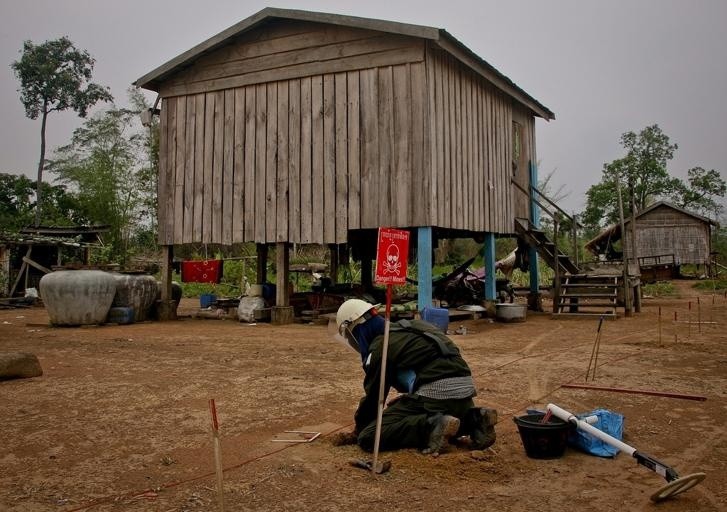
[422,414,460,455]
[470,408,496,450]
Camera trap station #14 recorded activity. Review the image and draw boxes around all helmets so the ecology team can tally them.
[337,298,382,338]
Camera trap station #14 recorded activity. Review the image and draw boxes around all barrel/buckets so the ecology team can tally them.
[512,413,578,459]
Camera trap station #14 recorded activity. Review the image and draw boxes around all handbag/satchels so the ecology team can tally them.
[577,408,625,456]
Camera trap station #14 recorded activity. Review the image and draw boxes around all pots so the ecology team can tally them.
[495,302,529,323]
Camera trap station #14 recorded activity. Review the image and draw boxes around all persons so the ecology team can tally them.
[332,297,500,456]
